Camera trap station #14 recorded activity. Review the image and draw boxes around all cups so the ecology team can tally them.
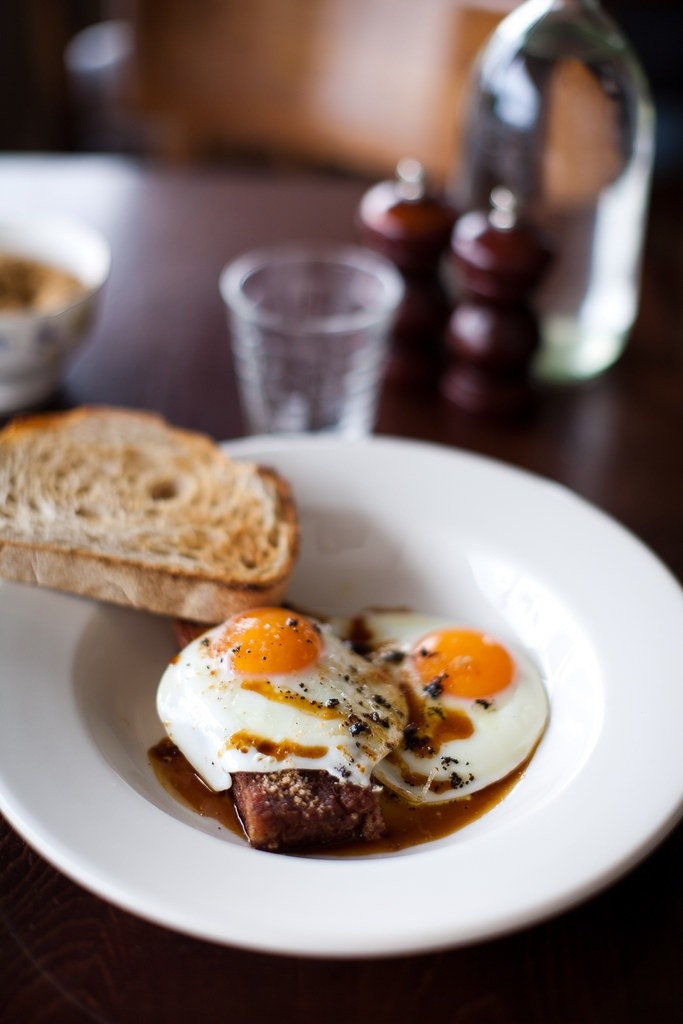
[220,243,403,437]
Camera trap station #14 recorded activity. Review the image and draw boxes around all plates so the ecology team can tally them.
[0,433,682,958]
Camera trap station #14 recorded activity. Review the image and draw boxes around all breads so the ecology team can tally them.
[1,405,300,621]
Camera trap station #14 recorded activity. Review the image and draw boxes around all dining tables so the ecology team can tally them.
[0,152,683,1024]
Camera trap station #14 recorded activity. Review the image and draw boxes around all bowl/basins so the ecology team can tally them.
[0,225,111,413]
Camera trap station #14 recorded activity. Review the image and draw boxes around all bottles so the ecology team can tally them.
[456,0,655,383]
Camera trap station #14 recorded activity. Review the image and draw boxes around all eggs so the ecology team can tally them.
[156,606,408,791]
[331,613,547,802]
